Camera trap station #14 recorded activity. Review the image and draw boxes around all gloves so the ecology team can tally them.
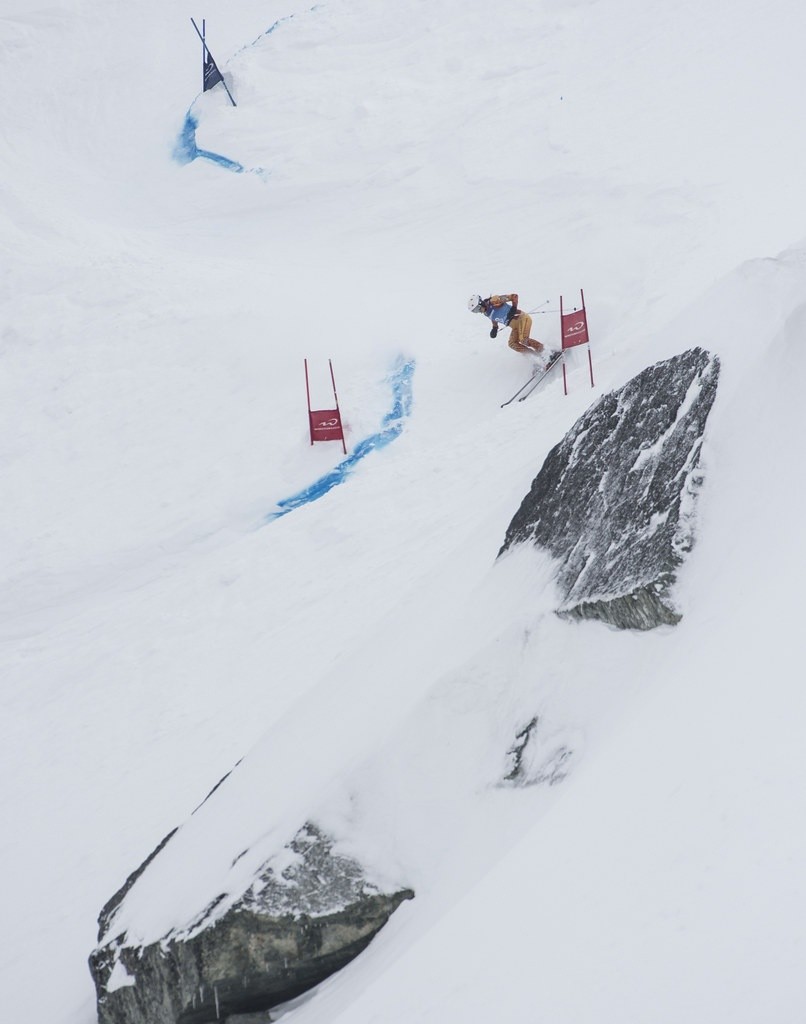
[507,306,516,319]
[490,328,496,338]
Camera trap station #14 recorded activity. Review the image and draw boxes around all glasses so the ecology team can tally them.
[473,304,482,314]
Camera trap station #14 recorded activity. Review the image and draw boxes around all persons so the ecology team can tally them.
[466,293,545,359]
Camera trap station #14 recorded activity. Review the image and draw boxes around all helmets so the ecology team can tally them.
[466,295,480,312]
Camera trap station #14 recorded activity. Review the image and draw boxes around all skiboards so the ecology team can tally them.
[501,350,562,408]
